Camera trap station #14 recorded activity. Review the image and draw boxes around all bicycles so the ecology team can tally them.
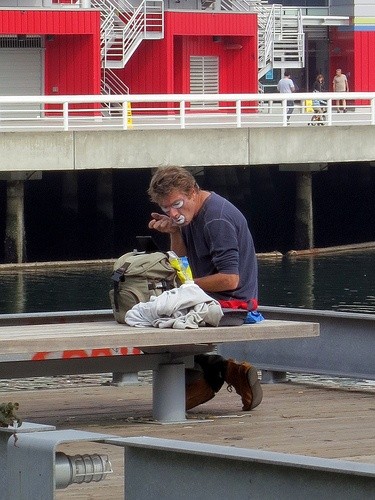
[308,107,327,126]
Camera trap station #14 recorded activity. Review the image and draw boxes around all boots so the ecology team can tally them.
[225,358,262,411]
[185,368,215,410]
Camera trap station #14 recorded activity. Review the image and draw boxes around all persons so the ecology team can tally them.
[311,74,327,121]
[333,69,349,113]
[277,71,295,125]
[148,166,264,412]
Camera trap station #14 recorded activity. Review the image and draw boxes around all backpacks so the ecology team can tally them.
[109,250,177,324]
